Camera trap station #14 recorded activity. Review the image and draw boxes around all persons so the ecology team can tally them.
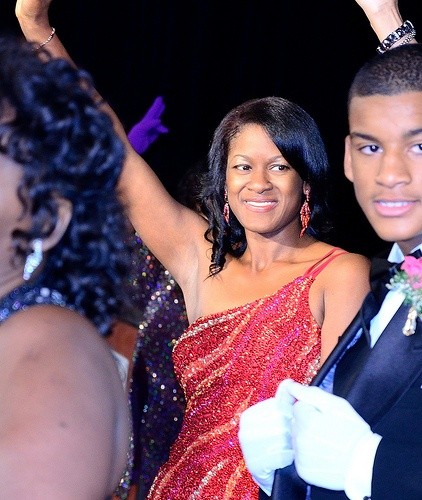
[238,43,422,500]
[354,0,422,57]
[0,41,189,500]
[14,1,372,500]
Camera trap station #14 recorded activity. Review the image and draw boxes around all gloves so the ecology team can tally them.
[287,381,383,498]
[237,378,297,496]
[128,96,170,153]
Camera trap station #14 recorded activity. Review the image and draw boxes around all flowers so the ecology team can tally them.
[385,255,422,336]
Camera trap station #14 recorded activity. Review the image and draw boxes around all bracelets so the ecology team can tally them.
[399,29,417,47]
[31,27,55,52]
[375,19,414,55]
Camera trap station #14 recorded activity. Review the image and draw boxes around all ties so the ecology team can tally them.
[332,251,422,399]
